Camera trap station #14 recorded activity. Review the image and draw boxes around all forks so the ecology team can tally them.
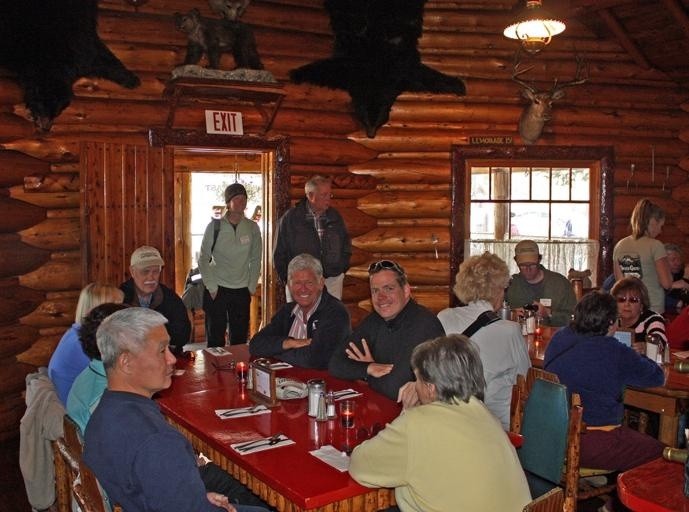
[219,403,261,416]
[234,431,285,449]
[225,407,270,417]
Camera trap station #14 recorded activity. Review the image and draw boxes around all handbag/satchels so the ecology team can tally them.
[182,268,205,309]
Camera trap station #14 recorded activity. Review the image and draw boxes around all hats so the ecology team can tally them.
[515,240,539,263]
[225,184,247,202]
[130,246,164,268]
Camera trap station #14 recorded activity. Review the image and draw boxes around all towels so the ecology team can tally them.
[308,444,351,472]
[270,361,293,371]
[230,434,296,456]
[333,388,364,401]
[215,405,272,420]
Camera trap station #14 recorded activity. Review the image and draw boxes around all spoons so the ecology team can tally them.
[240,439,282,452]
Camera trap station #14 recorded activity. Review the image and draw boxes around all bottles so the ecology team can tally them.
[527,310,536,335]
[520,317,528,336]
[307,419,337,450]
[314,391,328,422]
[500,299,511,323]
[643,335,670,366]
[327,390,338,419]
[246,364,254,389]
[307,379,327,418]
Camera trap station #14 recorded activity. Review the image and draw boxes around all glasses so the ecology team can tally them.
[368,260,403,273]
[617,297,639,303]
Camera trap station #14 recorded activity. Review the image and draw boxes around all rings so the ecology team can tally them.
[216,493,224,500]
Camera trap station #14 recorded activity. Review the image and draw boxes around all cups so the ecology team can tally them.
[535,317,544,339]
[340,400,355,428]
[342,428,357,446]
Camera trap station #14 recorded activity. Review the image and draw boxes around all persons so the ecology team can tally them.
[681,262,688,294]
[115,244,193,361]
[502,236,578,332]
[603,272,619,291]
[81,306,274,512]
[347,332,535,510]
[197,181,263,350]
[602,276,671,372]
[64,301,267,505]
[543,289,673,473]
[272,176,352,307]
[434,249,533,444]
[611,197,674,320]
[248,250,355,370]
[327,256,448,401]
[46,283,126,411]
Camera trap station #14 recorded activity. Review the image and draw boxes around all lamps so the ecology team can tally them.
[496,0,570,56]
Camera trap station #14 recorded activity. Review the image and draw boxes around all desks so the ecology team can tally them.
[615,455,689,512]
[517,327,689,449]
[150,345,403,512]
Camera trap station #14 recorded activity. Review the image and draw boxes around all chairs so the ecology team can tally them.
[509,377,618,512]
[517,367,617,500]
[521,487,564,512]
[62,414,85,459]
[50,437,123,512]
